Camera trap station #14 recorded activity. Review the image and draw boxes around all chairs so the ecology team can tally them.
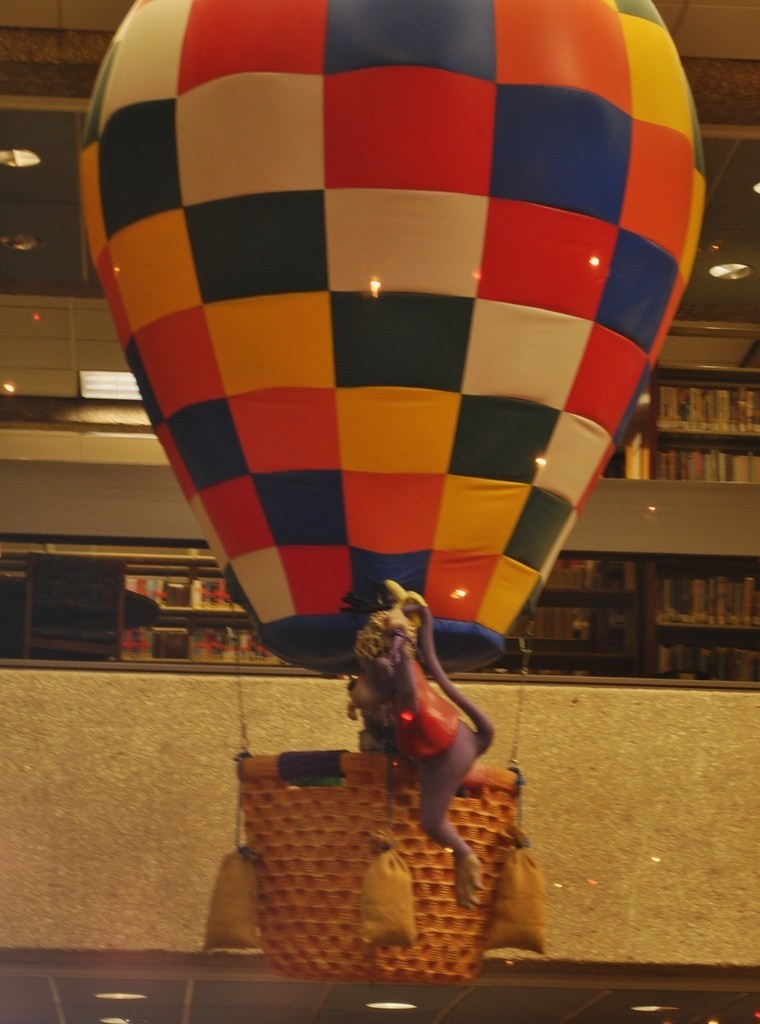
[25,553,130,662]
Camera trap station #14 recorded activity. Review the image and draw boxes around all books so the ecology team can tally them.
[126,577,279,664]
[521,363,760,684]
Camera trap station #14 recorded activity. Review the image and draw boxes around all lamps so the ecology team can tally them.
[79,371,143,402]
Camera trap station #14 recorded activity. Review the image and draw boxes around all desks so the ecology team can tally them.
[1,572,160,662]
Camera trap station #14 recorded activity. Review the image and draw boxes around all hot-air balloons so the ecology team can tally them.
[77,0,706,984]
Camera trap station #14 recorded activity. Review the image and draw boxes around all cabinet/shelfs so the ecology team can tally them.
[123,555,283,664]
[495,551,760,685]
[602,363,760,483]
